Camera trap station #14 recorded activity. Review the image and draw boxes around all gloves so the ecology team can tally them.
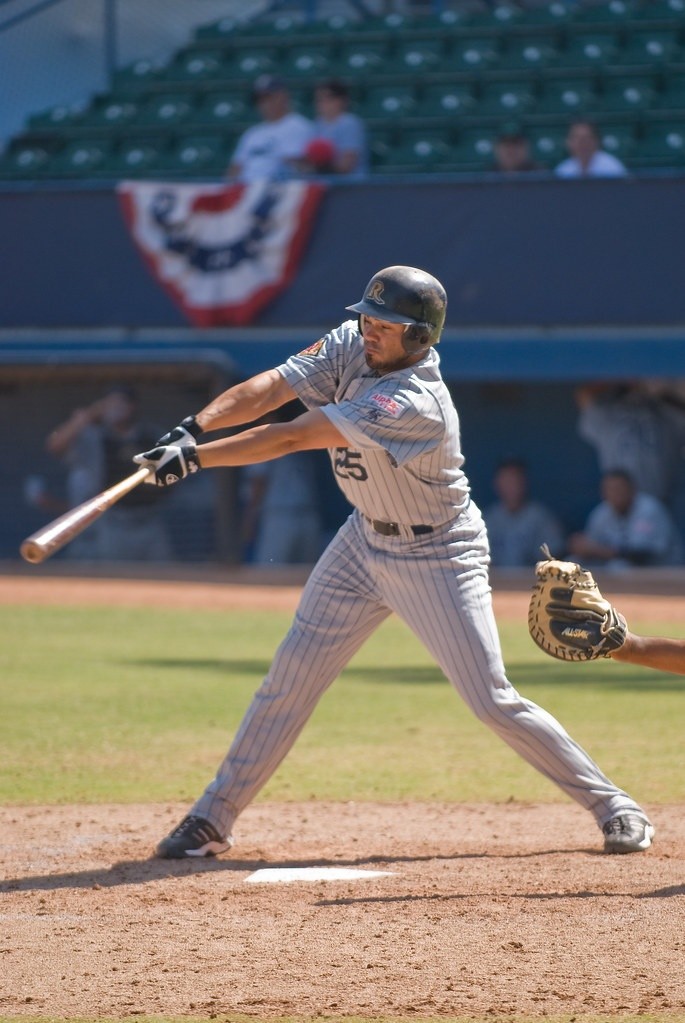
[132,415,204,487]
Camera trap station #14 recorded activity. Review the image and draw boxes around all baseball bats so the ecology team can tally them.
[19,462,158,565]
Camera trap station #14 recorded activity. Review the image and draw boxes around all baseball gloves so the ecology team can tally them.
[527,539,630,664]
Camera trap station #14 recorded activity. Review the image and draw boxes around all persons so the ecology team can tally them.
[553,118,632,176]
[475,456,571,562]
[134,265,659,858]
[527,543,685,678]
[224,70,379,188]
[41,374,322,565]
[571,379,685,565]
[479,120,541,172]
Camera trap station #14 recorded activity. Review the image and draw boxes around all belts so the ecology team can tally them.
[361,513,434,535]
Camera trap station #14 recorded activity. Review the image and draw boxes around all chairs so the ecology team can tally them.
[0,0,685,184]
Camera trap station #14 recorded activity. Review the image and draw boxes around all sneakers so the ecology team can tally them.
[155,815,233,857]
[602,812,655,853]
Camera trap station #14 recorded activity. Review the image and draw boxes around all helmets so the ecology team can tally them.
[345,264,447,354]
